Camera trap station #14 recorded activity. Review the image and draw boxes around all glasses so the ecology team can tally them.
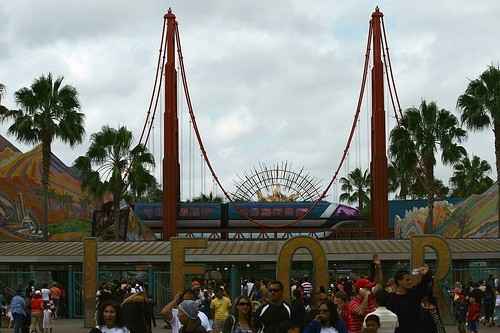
[318,309,330,313]
[237,302,250,306]
[268,288,282,292]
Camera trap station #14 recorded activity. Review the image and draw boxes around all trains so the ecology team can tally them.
[129,201,367,231]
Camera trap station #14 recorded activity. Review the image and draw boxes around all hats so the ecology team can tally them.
[47,304,51,306]
[355,278,376,290]
[387,278,396,285]
[34,290,42,296]
[178,299,199,319]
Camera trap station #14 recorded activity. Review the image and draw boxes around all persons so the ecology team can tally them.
[161,254,446,333]
[444,273,500,333]
[88,278,157,333]
[0,286,14,328]
[11,280,66,333]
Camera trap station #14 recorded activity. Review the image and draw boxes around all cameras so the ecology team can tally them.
[412,269,422,274]
